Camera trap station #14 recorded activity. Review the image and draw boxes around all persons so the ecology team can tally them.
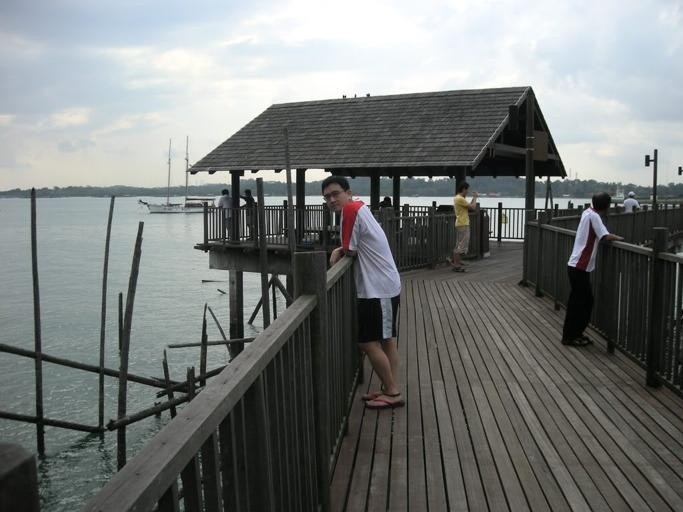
[321,176,405,409]
[218,189,232,235]
[561,192,624,346]
[450,180,478,271]
[238,189,255,241]
[623,191,640,214]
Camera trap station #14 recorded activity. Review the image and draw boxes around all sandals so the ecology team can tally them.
[452,265,467,273]
[562,333,590,347]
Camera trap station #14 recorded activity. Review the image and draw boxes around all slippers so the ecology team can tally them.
[362,387,404,408]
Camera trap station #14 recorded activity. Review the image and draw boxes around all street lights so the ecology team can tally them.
[677,165,683,175]
[644,148,660,210]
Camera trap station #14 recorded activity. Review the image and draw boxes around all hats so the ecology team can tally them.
[628,191,635,196]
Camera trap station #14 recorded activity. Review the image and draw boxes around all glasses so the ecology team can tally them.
[323,188,349,200]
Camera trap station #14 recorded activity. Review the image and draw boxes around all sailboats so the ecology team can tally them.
[136,137,220,216]
